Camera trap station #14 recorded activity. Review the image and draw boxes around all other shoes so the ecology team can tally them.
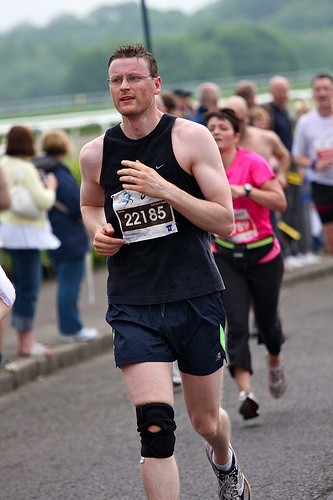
[171,360,183,384]
[59,328,97,343]
[283,252,321,273]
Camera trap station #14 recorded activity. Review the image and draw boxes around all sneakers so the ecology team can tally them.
[268,365,286,398]
[205,439,252,500]
[238,390,260,421]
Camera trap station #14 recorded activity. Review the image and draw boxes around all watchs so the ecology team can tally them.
[244,184,252,195]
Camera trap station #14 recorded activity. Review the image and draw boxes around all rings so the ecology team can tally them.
[132,177,134,182]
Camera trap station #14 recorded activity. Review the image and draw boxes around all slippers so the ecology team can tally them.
[18,343,54,357]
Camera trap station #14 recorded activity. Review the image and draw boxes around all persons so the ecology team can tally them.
[1,126,98,360]
[203,109,284,422]
[79,47,252,500]
[156,75,319,271]
[292,74,333,251]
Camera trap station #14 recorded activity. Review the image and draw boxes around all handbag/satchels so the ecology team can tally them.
[10,163,41,219]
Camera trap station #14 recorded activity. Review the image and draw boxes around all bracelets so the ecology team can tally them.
[312,159,317,169]
